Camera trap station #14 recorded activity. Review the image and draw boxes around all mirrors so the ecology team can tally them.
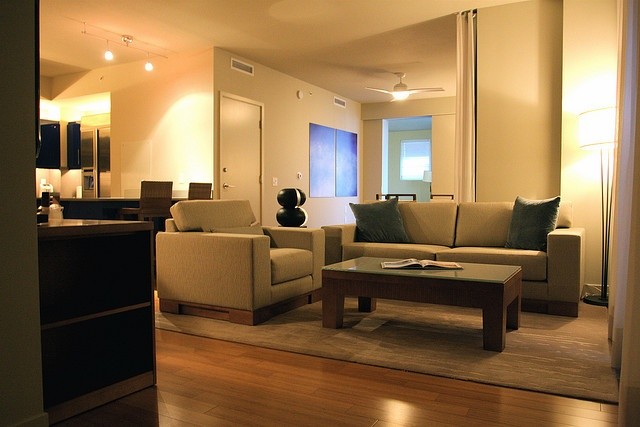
[53,92,111,199]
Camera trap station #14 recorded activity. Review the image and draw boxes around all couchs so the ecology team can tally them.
[321,201,584,318]
[156,199,325,326]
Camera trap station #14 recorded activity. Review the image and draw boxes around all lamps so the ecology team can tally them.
[103,39,114,61]
[579,108,616,304]
[144,52,156,72]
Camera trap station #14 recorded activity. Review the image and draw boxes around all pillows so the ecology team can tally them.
[351,201,408,243]
[504,196,563,250]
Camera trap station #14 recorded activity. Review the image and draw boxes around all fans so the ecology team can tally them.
[364,73,445,104]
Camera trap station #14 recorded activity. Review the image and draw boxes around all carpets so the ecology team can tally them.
[155,304,619,406]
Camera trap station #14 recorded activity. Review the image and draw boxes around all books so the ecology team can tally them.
[381,258,464,269]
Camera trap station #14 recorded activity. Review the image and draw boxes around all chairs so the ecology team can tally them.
[188,183,213,199]
[118,181,172,254]
